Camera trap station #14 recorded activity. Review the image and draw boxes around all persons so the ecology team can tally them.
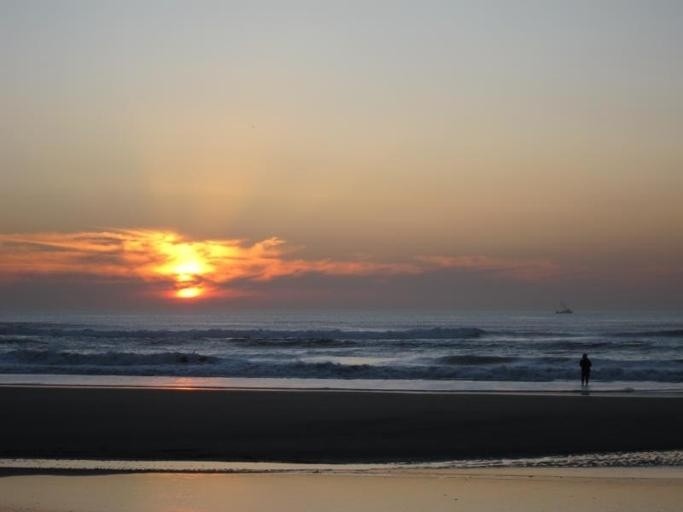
[579,353,591,386]
